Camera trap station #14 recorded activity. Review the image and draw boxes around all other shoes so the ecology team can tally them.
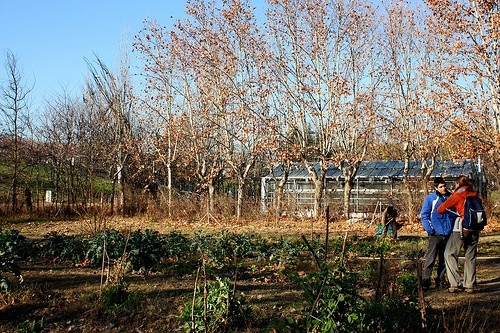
[447,287,462,292]
[423,286,429,292]
[435,286,448,288]
[465,289,473,293]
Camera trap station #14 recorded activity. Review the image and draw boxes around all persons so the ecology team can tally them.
[420,177,456,293]
[437,175,481,293]
[381,206,398,242]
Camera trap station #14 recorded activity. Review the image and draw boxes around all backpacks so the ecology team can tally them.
[456,193,487,232]
